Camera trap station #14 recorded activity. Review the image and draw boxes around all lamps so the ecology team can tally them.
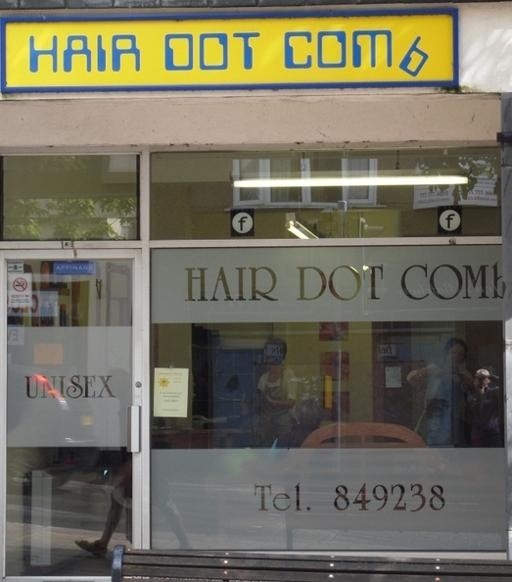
[287,219,321,239]
[230,150,468,188]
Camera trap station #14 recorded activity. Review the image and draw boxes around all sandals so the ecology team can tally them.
[74,538,107,558]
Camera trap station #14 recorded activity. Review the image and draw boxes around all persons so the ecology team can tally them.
[407,338,504,449]
[254,340,297,437]
[74,453,191,559]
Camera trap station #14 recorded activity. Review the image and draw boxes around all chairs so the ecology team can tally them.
[301,421,427,448]
